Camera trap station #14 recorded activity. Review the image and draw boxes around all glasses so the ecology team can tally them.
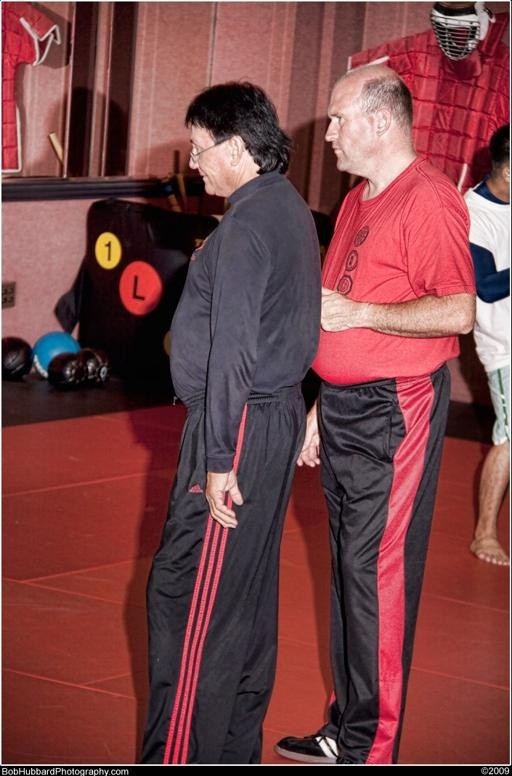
[189,137,230,163]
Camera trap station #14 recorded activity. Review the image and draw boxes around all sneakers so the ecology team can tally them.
[275,734,339,763]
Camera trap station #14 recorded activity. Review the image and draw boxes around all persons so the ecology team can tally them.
[137,76,320,763]
[275,63,480,766]
[458,121,511,566]
